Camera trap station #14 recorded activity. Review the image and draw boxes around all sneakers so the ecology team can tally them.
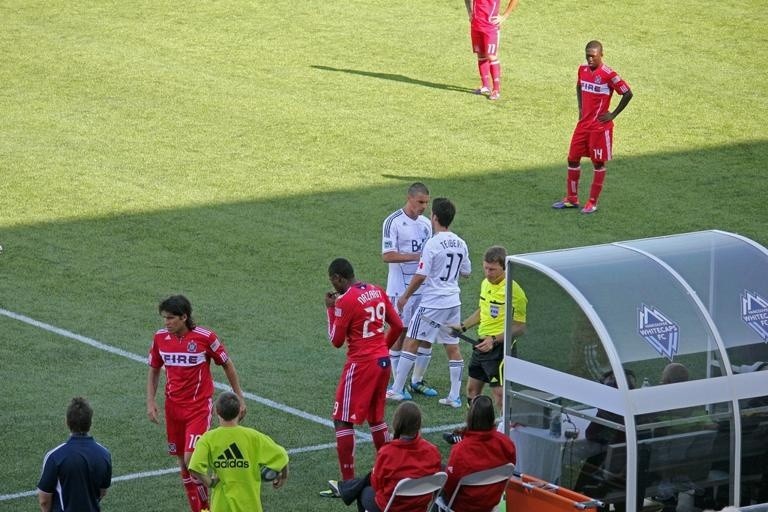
[438,397,462,408]
[552,199,578,209]
[476,87,489,94]
[581,202,597,213]
[319,488,335,497]
[385,389,404,401]
[409,380,438,396]
[442,432,463,444]
[403,388,413,400]
[328,479,342,497]
[489,90,500,100]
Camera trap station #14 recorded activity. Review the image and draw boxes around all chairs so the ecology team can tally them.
[364,462,516,512]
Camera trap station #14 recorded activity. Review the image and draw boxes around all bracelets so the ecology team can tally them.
[491,334,498,348]
[210,478,214,488]
[460,321,469,335]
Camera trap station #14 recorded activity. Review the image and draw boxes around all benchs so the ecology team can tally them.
[584,427,764,511]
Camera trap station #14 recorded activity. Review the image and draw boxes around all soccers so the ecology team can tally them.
[260,464,282,485]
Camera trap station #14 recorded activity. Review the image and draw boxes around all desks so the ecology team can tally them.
[498,408,606,486]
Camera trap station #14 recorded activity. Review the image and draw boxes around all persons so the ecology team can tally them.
[648,362,718,511]
[145,296,247,512]
[319,257,402,499]
[463,0,519,100]
[442,245,529,444]
[384,197,472,410]
[37,397,113,510]
[187,391,289,512]
[430,394,518,512]
[380,179,437,398]
[551,41,635,215]
[327,400,441,512]
[573,368,652,512]
[709,363,768,511]
